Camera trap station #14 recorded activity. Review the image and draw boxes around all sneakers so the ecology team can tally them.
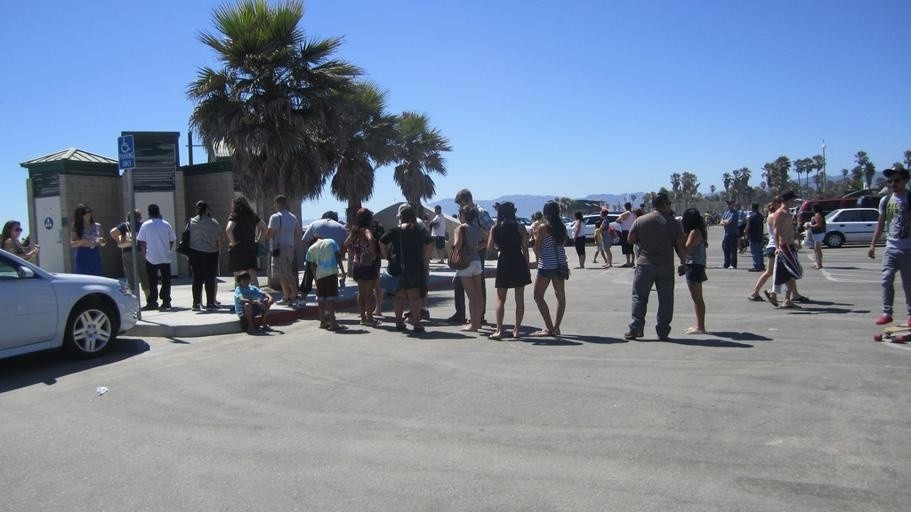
[624,330,643,339]
[876,317,892,324]
[275,297,305,309]
[192,301,220,311]
[395,309,429,332]
[141,305,171,312]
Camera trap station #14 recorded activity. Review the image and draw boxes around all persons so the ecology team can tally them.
[300,189,826,339]
[2,220,39,260]
[185,200,222,312]
[868,161,911,328]
[268,195,302,306]
[225,193,268,289]
[137,203,176,311]
[70,203,107,275]
[233,271,273,336]
[109,209,150,300]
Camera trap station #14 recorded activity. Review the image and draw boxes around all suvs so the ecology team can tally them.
[559,212,630,246]
[794,196,860,239]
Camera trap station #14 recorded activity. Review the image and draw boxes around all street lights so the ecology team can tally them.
[816,142,828,190]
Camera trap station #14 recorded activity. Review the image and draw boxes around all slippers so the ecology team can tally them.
[360,316,385,326]
[487,335,500,340]
[747,290,809,308]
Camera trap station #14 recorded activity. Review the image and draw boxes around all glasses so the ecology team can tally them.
[887,178,900,183]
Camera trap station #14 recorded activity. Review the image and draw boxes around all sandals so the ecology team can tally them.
[534,326,560,336]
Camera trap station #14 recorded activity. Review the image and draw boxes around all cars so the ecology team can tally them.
[858,195,884,208]
[803,207,890,249]
[0,248,143,375]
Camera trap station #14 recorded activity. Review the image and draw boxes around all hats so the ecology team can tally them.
[781,190,797,199]
[492,200,516,213]
[882,166,908,177]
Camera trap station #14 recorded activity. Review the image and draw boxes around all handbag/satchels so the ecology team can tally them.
[557,263,568,280]
[447,243,469,270]
[116,232,137,248]
[175,230,189,255]
[360,252,372,266]
[380,272,401,294]
